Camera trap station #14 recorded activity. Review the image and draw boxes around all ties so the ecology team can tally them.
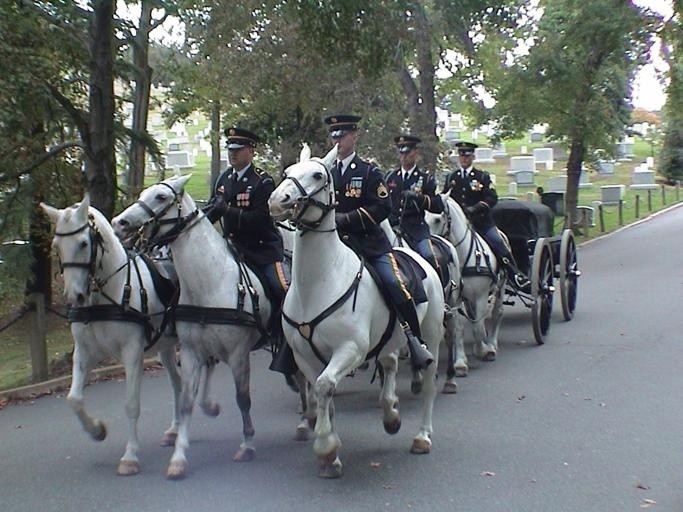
[231,170,240,186]
[402,171,410,184]
[336,160,345,177]
[462,168,469,179]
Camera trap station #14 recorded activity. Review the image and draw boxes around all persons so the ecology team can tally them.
[440,142,535,290]
[201,123,291,338]
[375,131,449,291]
[319,114,434,371]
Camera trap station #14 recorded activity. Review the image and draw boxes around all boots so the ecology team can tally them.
[397,299,433,366]
[500,253,530,289]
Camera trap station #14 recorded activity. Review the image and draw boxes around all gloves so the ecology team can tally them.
[210,194,230,214]
[402,189,419,202]
[334,211,350,230]
[466,202,486,218]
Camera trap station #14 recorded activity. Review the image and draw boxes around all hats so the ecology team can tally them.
[223,127,261,150]
[455,141,478,156]
[393,135,421,154]
[324,114,363,137]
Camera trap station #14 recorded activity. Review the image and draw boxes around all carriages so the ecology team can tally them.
[39,141,581,481]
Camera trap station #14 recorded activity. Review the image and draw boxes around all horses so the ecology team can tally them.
[110,173,309,478]
[38,192,221,476]
[267,141,450,476]
[428,181,513,375]
[372,209,465,401]
